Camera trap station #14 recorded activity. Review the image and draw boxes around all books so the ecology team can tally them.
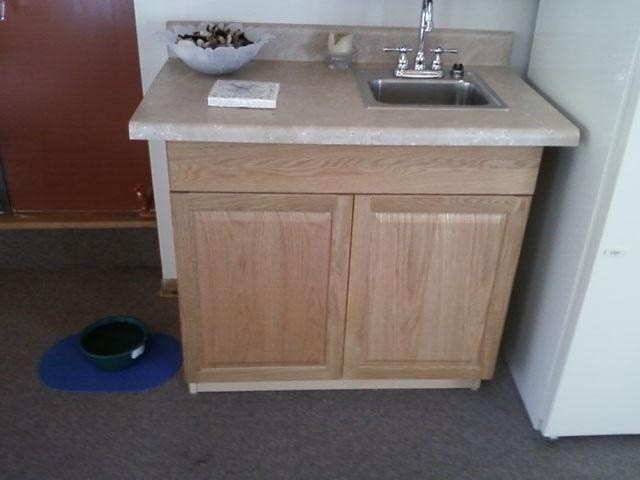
[207,78,282,110]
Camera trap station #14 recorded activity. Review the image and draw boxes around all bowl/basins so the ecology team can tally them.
[161,21,272,76]
[77,315,151,373]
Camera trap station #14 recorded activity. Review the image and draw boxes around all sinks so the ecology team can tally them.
[354,64,512,111]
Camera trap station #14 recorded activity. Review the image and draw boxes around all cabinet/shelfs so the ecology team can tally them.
[164,141,544,393]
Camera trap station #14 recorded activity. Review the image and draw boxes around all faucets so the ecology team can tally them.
[413,0,434,71]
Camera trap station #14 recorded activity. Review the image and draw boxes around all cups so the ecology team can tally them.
[132,186,152,209]
